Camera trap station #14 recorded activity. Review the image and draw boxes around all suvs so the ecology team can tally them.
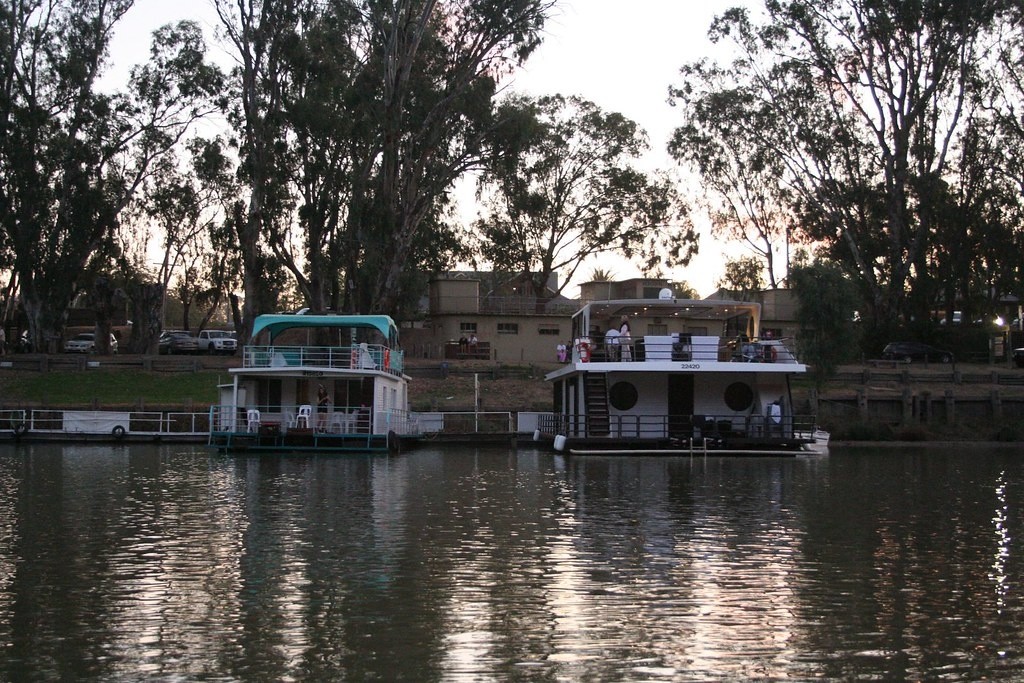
[883,342,952,364]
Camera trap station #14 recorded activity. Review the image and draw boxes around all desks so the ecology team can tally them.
[259,421,282,437]
[755,339,782,361]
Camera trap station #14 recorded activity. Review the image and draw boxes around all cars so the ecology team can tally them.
[63,332,118,356]
[0,336,35,355]
[158,329,198,356]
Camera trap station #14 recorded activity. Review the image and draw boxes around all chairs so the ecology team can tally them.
[247,408,261,433]
[327,409,359,434]
[285,405,312,429]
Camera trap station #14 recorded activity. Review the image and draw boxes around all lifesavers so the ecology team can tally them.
[111,426,125,439]
[14,423,27,436]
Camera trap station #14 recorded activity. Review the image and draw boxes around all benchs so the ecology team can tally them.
[447,340,490,360]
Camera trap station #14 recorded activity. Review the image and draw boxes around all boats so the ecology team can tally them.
[209,314,415,455]
[535,297,817,451]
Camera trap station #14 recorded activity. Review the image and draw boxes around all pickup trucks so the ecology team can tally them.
[194,329,238,355]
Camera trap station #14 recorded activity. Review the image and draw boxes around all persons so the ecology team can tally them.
[731,329,749,351]
[316,384,332,434]
[603,315,632,362]
[0,326,6,356]
[458,333,480,355]
[557,339,574,362]
